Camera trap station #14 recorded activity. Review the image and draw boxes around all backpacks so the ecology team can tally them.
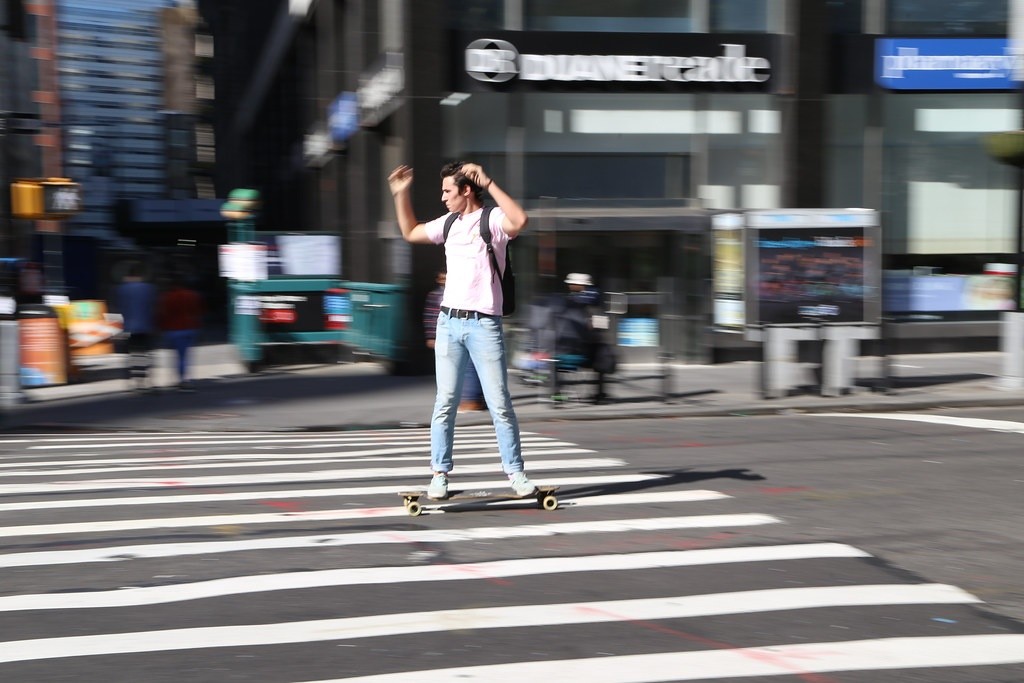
[443,206,516,316]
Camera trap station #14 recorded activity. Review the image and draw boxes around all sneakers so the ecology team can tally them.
[508,471,537,497]
[426,472,448,500]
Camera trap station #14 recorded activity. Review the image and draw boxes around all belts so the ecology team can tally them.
[439,306,492,318]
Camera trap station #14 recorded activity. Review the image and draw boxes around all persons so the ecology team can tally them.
[155,276,202,392]
[387,161,541,500]
[546,272,607,400]
[423,268,484,410]
[112,260,158,393]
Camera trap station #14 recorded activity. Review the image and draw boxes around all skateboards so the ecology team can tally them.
[397,485,560,517]
